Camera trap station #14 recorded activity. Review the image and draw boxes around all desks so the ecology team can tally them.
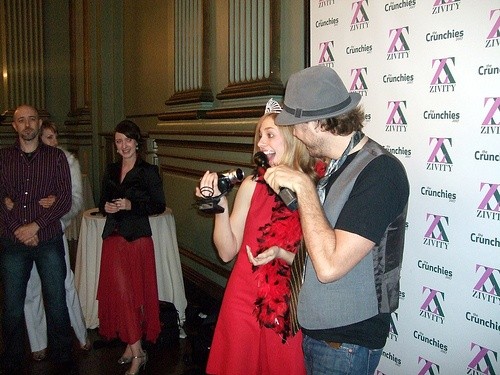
[74,206,188,339]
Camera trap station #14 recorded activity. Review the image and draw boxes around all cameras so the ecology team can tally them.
[198,169,245,213]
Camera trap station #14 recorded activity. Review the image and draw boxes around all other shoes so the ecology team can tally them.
[33,348,47,360]
[81,340,91,350]
[63,354,78,375]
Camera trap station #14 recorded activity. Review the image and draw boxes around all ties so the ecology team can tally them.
[289,136,367,337]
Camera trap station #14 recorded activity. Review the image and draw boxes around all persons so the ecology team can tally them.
[95,119,167,375]
[5,119,90,361]
[0,106,73,375]
[195,98,328,375]
[265,66,410,375]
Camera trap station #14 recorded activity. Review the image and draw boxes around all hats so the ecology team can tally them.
[275,66,361,126]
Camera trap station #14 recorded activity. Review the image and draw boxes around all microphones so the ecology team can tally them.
[253,152,298,210]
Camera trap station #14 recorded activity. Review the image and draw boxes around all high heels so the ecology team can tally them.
[126,350,148,375]
[119,352,133,364]
